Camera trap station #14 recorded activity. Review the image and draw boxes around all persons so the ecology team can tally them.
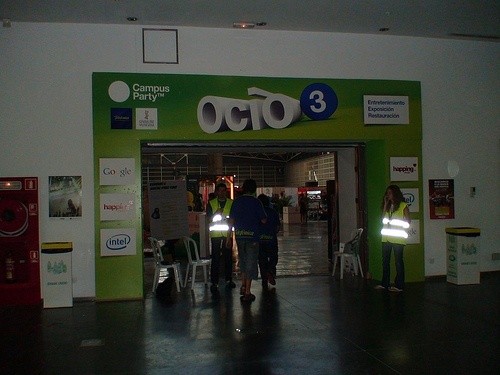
[228,179,267,299]
[299,193,308,223]
[375,184,411,292]
[257,193,280,288]
[205,182,236,296]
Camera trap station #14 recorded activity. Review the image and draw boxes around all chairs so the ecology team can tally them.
[182,236,210,289]
[331,228,365,279]
[148,236,183,293]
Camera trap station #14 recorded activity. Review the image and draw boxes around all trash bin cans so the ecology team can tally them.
[446,228,480,285]
[40,241,74,308]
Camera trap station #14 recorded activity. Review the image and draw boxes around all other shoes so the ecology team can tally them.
[240,285,256,301]
[262,281,268,287]
[375,285,405,293]
[267,272,276,285]
[210,281,236,292]
